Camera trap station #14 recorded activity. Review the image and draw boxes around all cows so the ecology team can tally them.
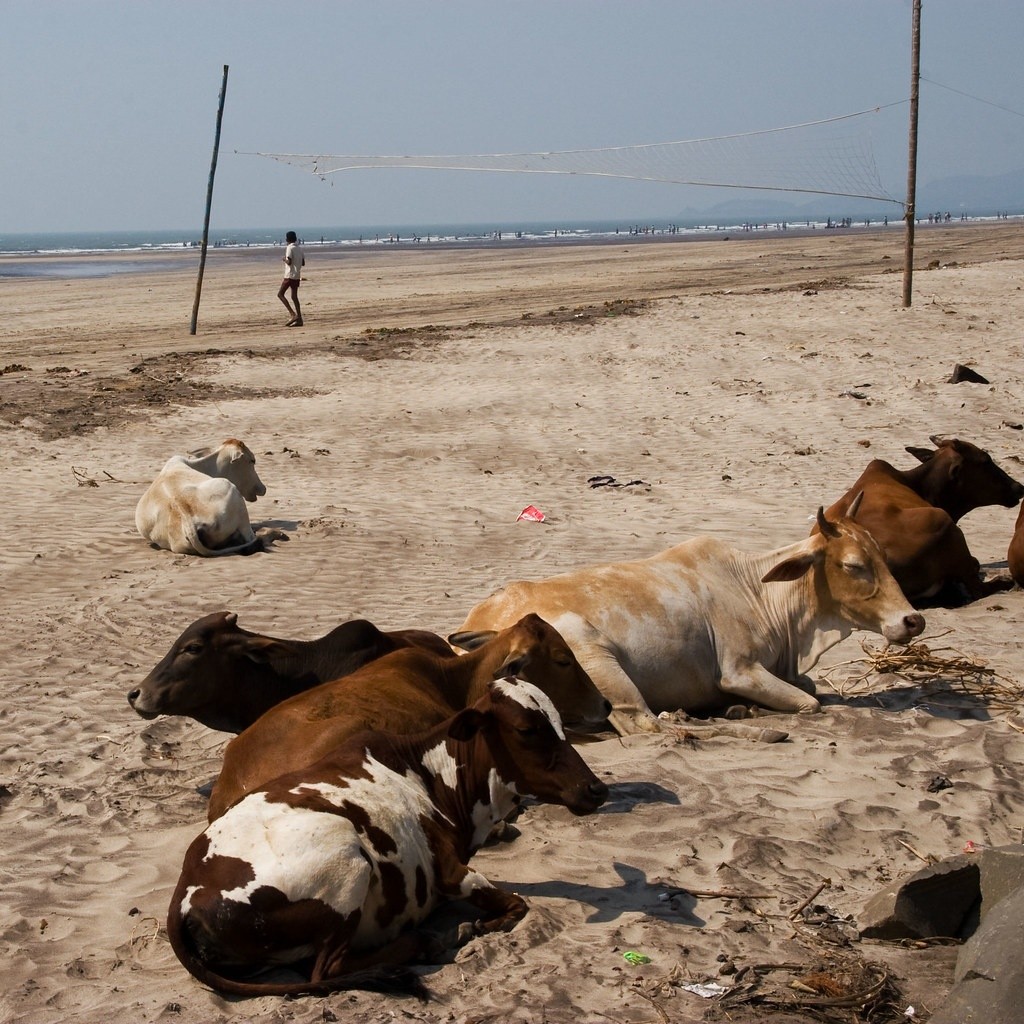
[126,608,608,996]
[136,438,291,558]
[810,435,1024,608]
[444,487,928,741]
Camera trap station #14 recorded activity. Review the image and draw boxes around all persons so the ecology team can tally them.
[277,231,306,326]
[928,211,967,224]
[397,234,399,241]
[616,220,815,236]
[998,210,1007,220]
[411,230,522,241]
[376,234,379,242]
[298,238,304,244]
[864,218,870,228]
[883,216,887,225]
[390,233,393,242]
[183,240,203,246]
[554,228,557,237]
[280,239,282,245]
[273,240,276,245]
[247,240,250,246]
[213,239,236,247]
[825,217,852,229]
[321,237,323,243]
